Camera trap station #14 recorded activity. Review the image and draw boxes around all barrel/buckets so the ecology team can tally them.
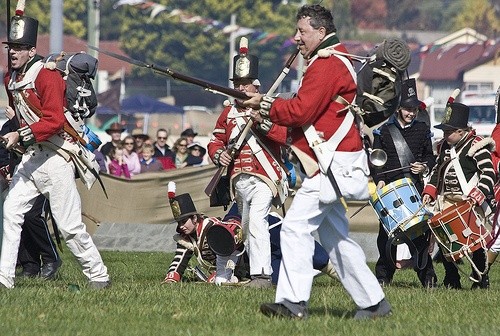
[284,162,296,188]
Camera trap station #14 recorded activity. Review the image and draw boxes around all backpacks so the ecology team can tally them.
[301,47,404,148]
[8,51,98,139]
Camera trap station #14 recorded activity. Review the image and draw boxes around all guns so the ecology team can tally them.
[205,42,301,196]
[83,44,253,103]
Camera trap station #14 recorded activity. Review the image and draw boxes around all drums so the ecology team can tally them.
[208,216,244,256]
[427,200,492,263]
[369,177,433,243]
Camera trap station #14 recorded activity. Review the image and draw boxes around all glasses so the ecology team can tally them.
[157,136,167,140]
[191,148,201,152]
[179,144,186,147]
[124,142,134,145]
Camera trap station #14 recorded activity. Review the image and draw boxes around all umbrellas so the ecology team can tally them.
[96,94,184,134]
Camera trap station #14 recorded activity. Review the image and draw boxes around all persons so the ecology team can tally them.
[180,128,198,146]
[138,144,164,173]
[100,122,127,160]
[421,102,495,292]
[0,14,109,291]
[484,85,500,274]
[150,128,177,169]
[368,78,436,289]
[108,146,132,179]
[183,143,206,167]
[207,53,290,288]
[164,195,344,288]
[131,129,151,159]
[235,4,392,320]
[169,138,190,169]
[118,131,142,176]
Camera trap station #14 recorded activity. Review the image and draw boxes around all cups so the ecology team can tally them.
[368,147,387,170]
[85,131,102,152]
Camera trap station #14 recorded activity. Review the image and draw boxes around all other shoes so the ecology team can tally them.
[469,263,492,280]
[87,280,110,292]
[354,298,393,323]
[441,280,461,289]
[40,259,62,279]
[470,282,490,290]
[261,301,311,321]
[246,276,272,289]
[16,269,38,279]
[0,282,5,289]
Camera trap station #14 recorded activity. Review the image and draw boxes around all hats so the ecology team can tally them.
[105,122,125,134]
[181,129,198,137]
[167,181,200,223]
[398,78,421,107]
[187,142,206,156]
[206,214,243,256]
[230,37,260,85]
[433,88,473,132]
[130,128,149,142]
[2,0,40,50]
